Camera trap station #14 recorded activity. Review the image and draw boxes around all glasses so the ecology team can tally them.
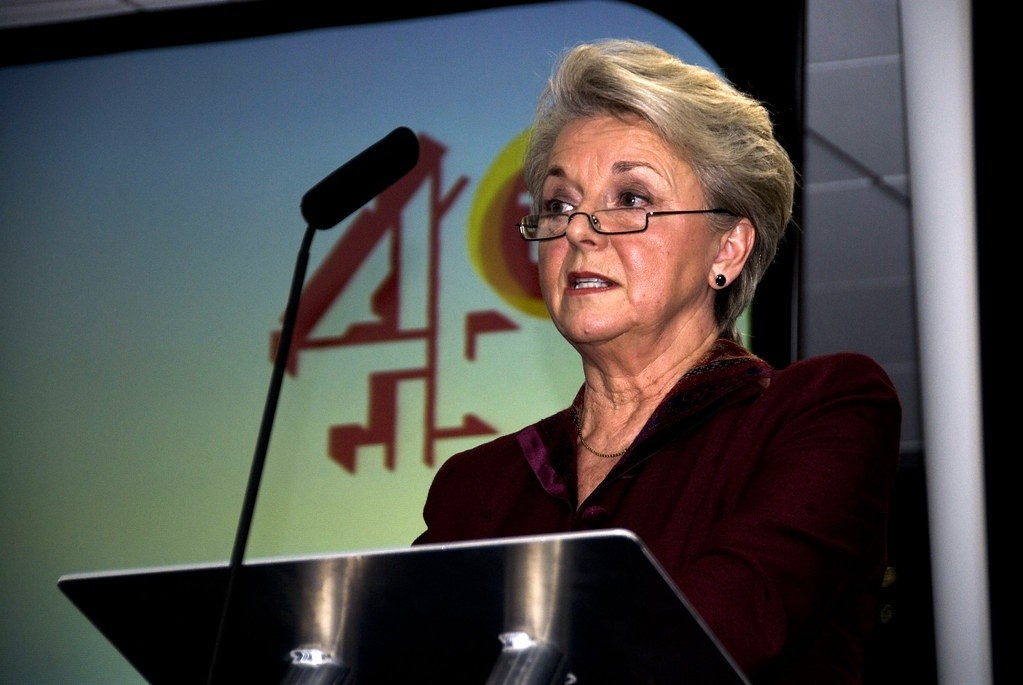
[515,205,743,241]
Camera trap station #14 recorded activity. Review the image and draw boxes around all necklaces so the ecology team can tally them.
[579,408,627,459]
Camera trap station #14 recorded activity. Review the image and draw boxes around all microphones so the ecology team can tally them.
[207,127,421,685]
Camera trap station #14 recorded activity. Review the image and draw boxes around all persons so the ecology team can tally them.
[412,39,901,685]
